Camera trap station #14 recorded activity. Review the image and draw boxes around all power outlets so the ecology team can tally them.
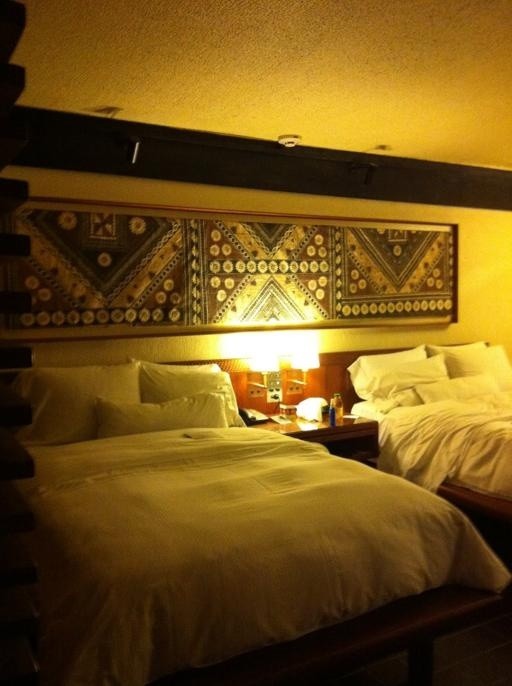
[247,372,265,398]
[287,369,303,395]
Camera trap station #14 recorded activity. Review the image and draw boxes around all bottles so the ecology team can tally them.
[330,398,335,425]
[333,393,344,424]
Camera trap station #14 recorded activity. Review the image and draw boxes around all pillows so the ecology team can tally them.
[346,340,512,414]
[11,356,249,447]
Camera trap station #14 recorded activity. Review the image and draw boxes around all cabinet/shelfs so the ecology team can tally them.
[0,0,40,686]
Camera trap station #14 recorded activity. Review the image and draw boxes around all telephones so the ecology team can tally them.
[240,408,269,424]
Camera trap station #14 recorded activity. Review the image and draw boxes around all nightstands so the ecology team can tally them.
[247,412,378,470]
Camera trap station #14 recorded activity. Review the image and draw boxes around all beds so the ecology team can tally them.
[319,341,512,525]
[1,356,512,686]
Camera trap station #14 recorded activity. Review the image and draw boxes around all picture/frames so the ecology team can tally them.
[0,197,459,345]
